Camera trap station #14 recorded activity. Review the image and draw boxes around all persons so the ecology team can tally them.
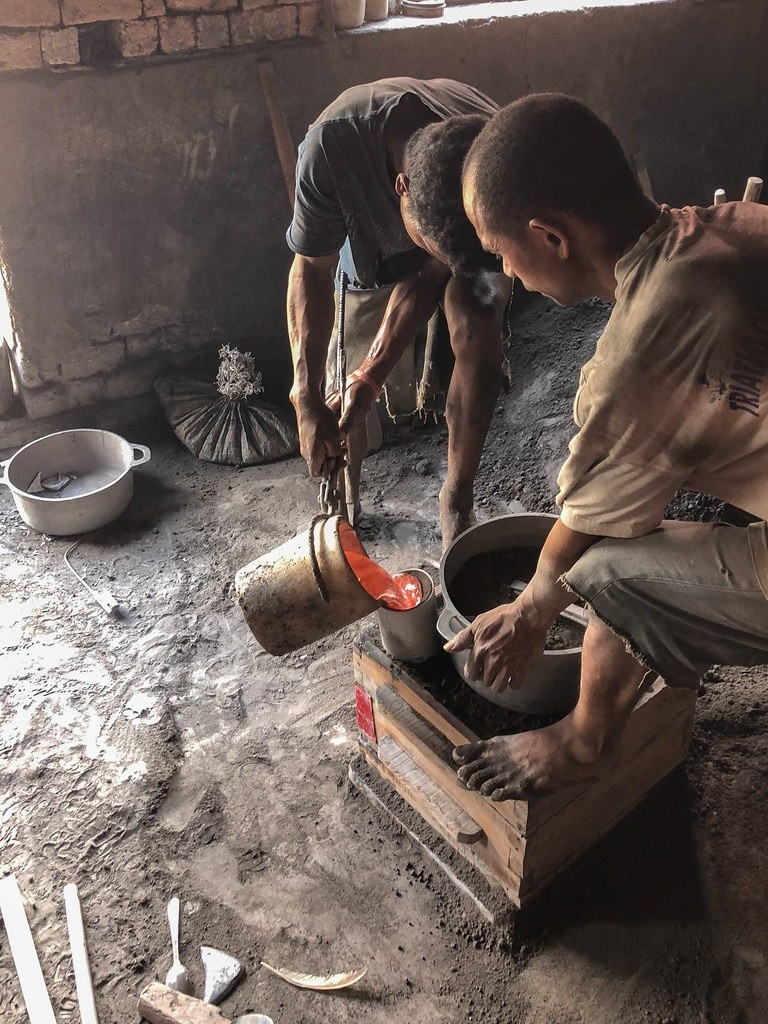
[286,77,509,562]
[441,88,768,803]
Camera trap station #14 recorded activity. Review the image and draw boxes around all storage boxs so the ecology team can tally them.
[353,584,699,906]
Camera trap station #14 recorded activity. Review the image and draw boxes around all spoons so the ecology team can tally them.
[165,896,190,993]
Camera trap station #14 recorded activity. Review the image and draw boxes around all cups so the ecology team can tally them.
[377,568,436,665]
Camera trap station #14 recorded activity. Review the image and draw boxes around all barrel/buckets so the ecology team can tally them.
[235,515,380,656]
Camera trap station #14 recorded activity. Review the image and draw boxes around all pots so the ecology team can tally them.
[439,511,584,715]
[0,427,150,536]
